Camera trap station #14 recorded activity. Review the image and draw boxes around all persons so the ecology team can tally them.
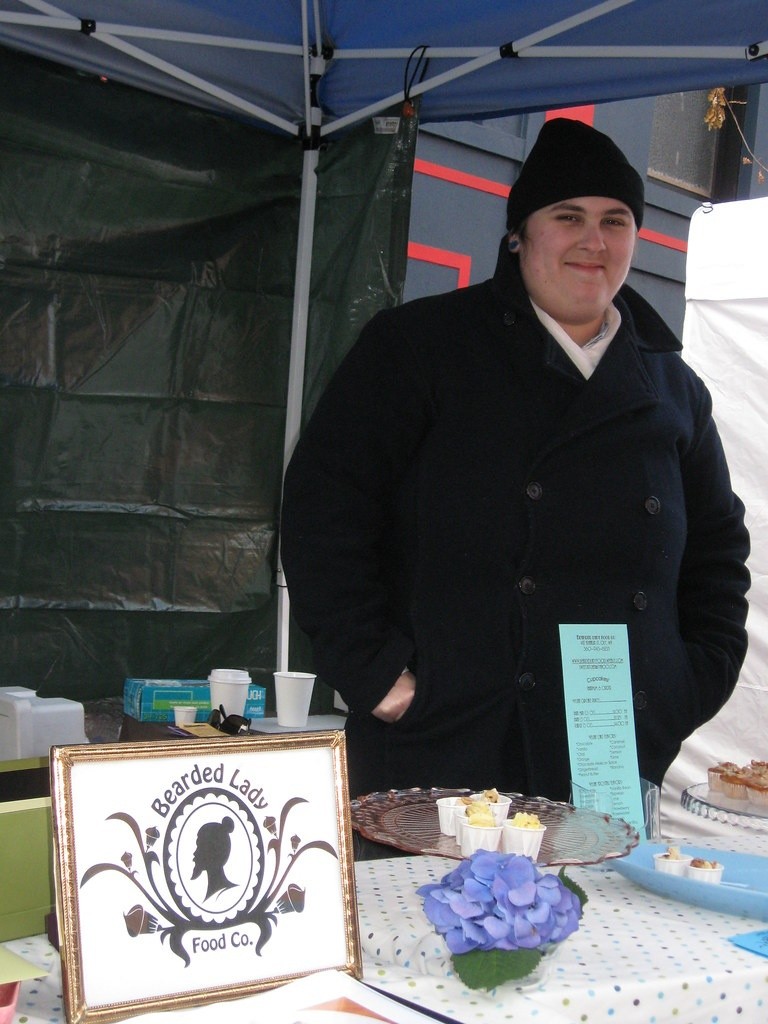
[278,119,750,803]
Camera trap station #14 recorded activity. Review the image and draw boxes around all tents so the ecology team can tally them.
[0,0,768,672]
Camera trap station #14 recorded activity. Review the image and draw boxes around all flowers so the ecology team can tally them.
[415,848,589,992]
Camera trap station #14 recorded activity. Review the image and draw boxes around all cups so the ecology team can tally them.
[273,671,317,727]
[207,669,252,724]
[653,853,693,876]
[436,793,547,864]
[686,863,724,884]
[173,706,198,727]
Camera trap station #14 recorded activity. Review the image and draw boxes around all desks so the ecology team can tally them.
[0,832,768,1024]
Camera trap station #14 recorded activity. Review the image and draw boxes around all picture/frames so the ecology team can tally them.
[51,728,362,1024]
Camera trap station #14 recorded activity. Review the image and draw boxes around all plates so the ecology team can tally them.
[681,782,768,834]
[349,787,640,865]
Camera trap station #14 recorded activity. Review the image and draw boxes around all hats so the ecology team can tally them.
[506,117,646,231]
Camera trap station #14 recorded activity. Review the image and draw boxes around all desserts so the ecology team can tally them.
[435,787,546,862]
[706,758,768,808]
[651,845,724,885]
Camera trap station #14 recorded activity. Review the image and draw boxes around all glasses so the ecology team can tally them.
[206,704,251,736]
